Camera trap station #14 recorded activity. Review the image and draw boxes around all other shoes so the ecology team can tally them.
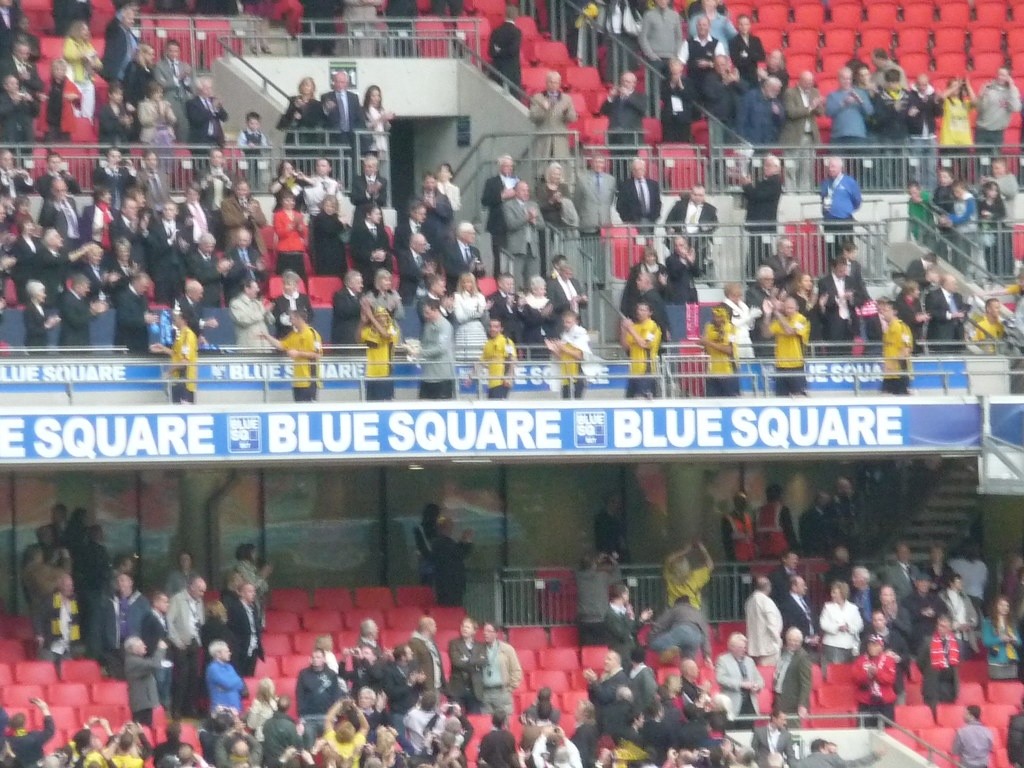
[660,645,681,664]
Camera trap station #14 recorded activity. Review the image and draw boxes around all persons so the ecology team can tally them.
[692,300,744,399]
[475,315,517,400]
[872,300,915,396]
[761,293,812,401]
[616,300,666,399]
[354,297,402,404]
[403,296,457,404]
[0,456,1024,767]
[254,306,324,402]
[165,308,201,405]
[0,1,1023,353]
[541,308,593,397]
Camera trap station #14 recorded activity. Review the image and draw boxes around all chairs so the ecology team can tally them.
[1,586,1024,768]
[0,1,1024,357]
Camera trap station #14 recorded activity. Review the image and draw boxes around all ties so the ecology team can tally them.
[204,98,214,118]
[522,204,531,239]
[595,173,602,187]
[737,659,745,681]
[905,567,919,592]
[336,90,346,132]
[949,294,958,315]
[419,255,424,269]
[5,172,10,184]
[172,61,176,79]
[241,248,247,262]
[463,246,471,264]
[130,222,136,235]
[797,597,810,620]
[125,26,132,54]
[689,205,699,224]
[638,178,648,208]
[193,202,207,235]
[20,63,25,73]
[322,180,328,195]
[417,226,423,234]
[60,198,76,234]
